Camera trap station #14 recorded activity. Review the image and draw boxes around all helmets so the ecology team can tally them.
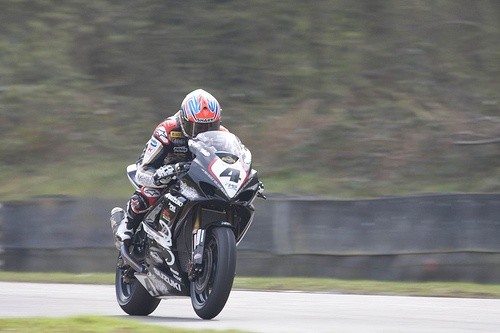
[179,89,222,139]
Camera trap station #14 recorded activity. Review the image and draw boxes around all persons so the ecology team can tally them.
[114,89,230,242]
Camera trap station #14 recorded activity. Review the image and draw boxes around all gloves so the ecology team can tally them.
[153,164,176,186]
[257,180,264,196]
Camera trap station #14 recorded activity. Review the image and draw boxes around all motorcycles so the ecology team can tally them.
[110,130,267,319]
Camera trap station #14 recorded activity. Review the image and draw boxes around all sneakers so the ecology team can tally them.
[116,212,137,242]
